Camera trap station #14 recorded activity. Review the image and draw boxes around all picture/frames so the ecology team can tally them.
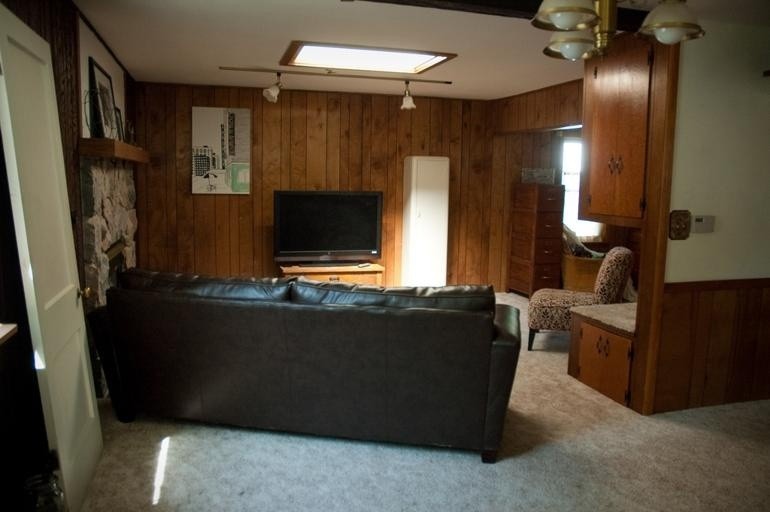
[88,56,121,141]
[115,106,125,143]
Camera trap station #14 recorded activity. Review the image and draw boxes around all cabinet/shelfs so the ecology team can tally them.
[504,182,565,302]
[579,38,654,229]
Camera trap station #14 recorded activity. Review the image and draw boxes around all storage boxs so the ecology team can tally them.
[560,242,605,292]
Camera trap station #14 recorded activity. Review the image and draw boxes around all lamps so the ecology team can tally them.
[262,72,283,103]
[400,81,417,110]
[530,0,706,64]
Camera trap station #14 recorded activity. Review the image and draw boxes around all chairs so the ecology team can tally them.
[527,245,636,351]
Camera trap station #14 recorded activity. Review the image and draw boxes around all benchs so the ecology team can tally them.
[569,302,637,408]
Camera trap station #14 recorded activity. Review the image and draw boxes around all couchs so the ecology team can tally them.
[85,267,521,464]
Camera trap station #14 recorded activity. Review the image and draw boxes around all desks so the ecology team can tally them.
[0,323,17,344]
[279,261,385,286]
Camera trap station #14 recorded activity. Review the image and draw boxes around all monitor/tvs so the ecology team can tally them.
[273,191,383,267]
